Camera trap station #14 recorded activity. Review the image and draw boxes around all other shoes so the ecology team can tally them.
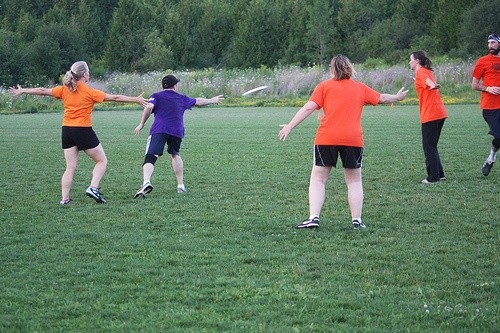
[422,176,446,184]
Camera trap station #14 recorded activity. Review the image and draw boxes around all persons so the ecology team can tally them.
[133,74,224,199]
[8,61,151,205]
[408,50,448,184]
[472,33,500,177]
[277,53,409,229]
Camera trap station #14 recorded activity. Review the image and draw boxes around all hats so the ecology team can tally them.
[487,33,500,43]
[162,75,180,89]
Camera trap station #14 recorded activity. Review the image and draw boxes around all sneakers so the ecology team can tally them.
[86,187,106,203]
[177,184,185,194]
[352,219,366,229]
[296,217,319,228]
[133,181,153,200]
[481,154,495,176]
[60,199,73,204]
